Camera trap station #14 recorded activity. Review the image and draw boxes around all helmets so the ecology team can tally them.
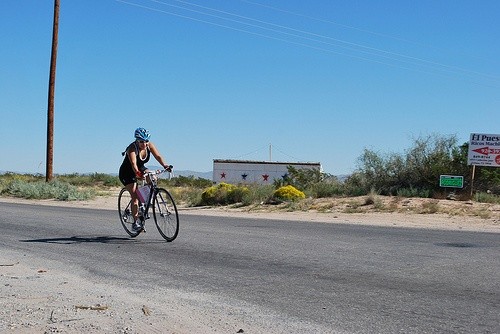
[134,127,151,140]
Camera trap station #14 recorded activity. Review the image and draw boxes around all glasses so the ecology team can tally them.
[137,139,150,143]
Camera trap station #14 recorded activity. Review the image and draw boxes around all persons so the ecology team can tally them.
[119,127,172,229]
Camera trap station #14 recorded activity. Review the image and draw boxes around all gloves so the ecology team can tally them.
[165,164,174,172]
[134,170,144,180]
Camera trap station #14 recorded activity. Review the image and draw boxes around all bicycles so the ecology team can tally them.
[117,167,180,242]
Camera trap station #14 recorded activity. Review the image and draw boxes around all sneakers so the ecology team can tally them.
[131,221,142,231]
[142,206,150,219]
